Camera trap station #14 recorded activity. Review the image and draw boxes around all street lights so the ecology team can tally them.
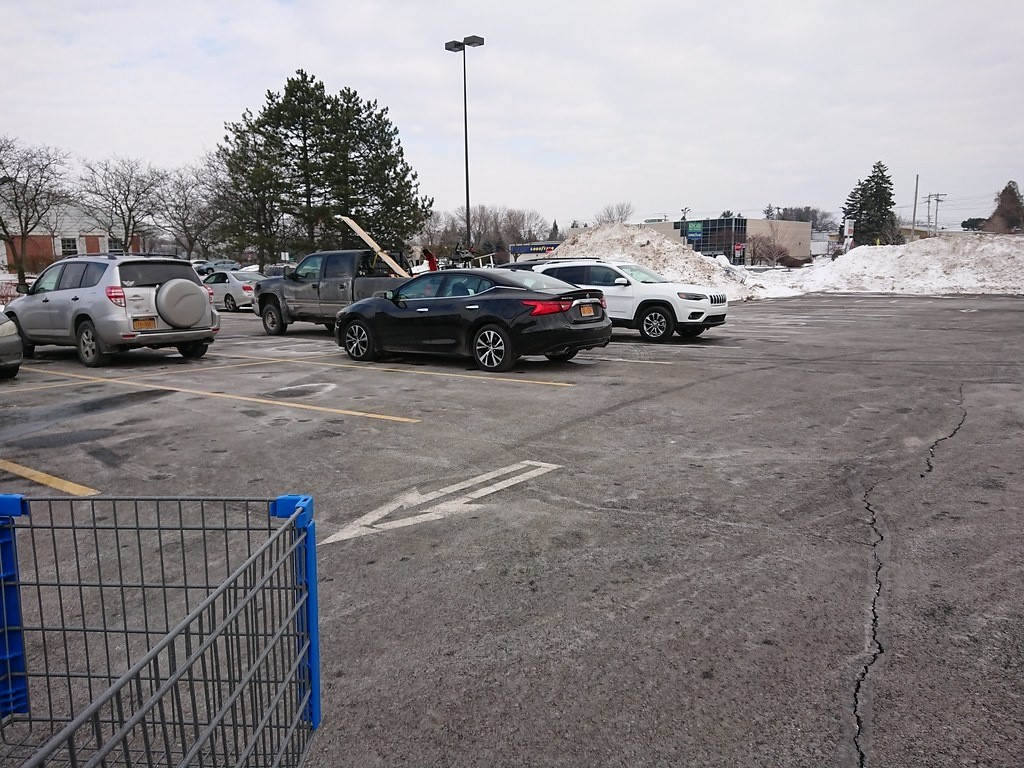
[445,34,485,249]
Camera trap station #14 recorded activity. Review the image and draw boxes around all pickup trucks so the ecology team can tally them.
[254,249,436,336]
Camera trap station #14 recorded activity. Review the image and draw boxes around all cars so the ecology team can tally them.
[334,269,613,373]
[203,271,269,311]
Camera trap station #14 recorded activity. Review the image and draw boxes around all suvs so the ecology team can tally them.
[532,260,728,343]
[189,259,210,276]
[3,251,222,368]
[201,259,242,276]
